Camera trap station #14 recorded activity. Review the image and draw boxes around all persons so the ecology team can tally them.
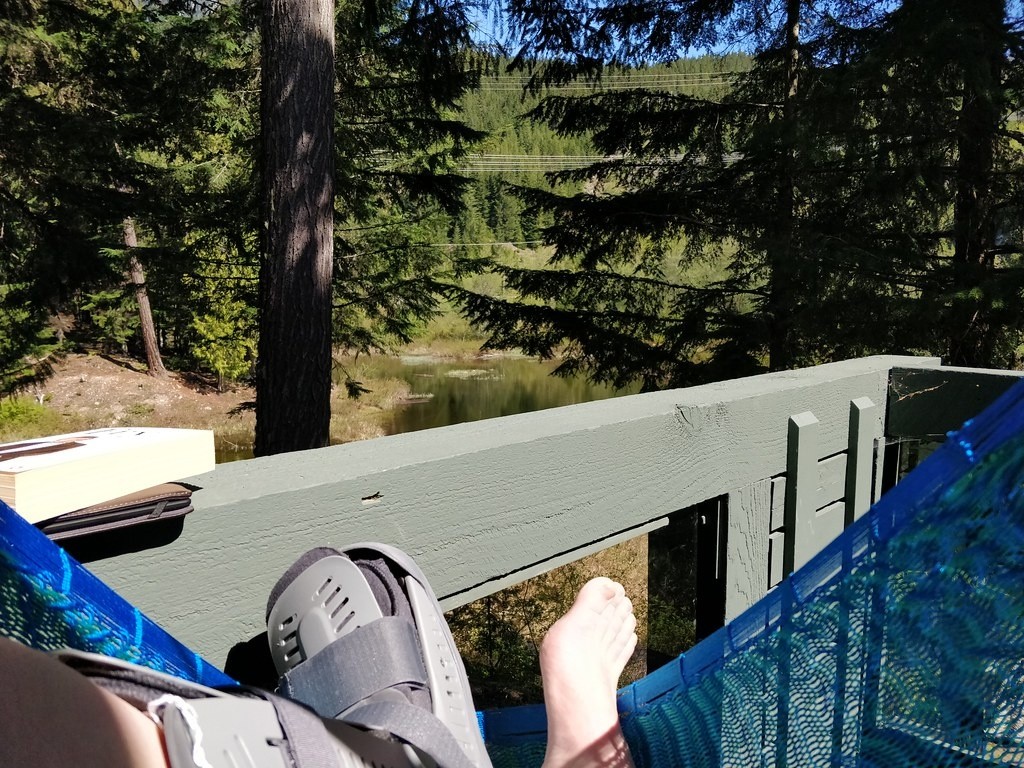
[0,542,638,768]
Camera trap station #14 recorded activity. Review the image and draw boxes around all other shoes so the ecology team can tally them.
[47,541,492,768]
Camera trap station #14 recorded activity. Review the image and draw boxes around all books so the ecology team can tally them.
[0,427,215,541]
